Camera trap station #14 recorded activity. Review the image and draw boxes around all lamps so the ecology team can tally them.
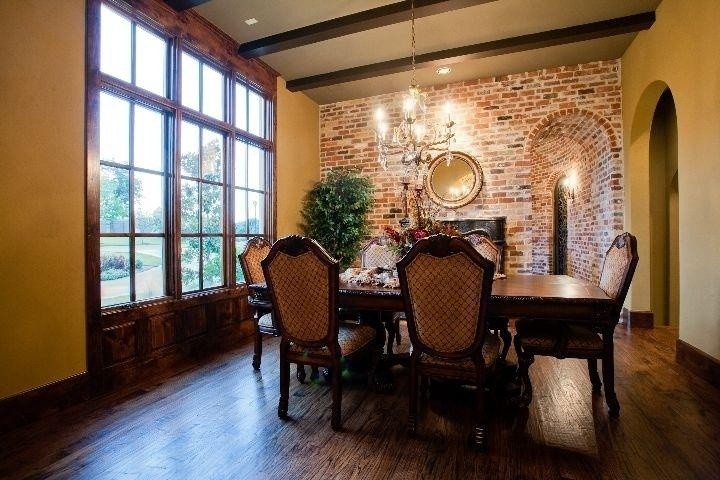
[370,0,452,178]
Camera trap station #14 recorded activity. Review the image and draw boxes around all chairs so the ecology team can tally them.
[239,236,277,369]
[396,233,505,420]
[515,234,637,413]
[262,234,379,429]
[359,229,502,275]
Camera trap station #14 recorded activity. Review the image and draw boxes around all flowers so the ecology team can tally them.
[383,221,459,259]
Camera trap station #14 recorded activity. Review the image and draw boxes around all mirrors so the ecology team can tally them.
[425,150,484,209]
